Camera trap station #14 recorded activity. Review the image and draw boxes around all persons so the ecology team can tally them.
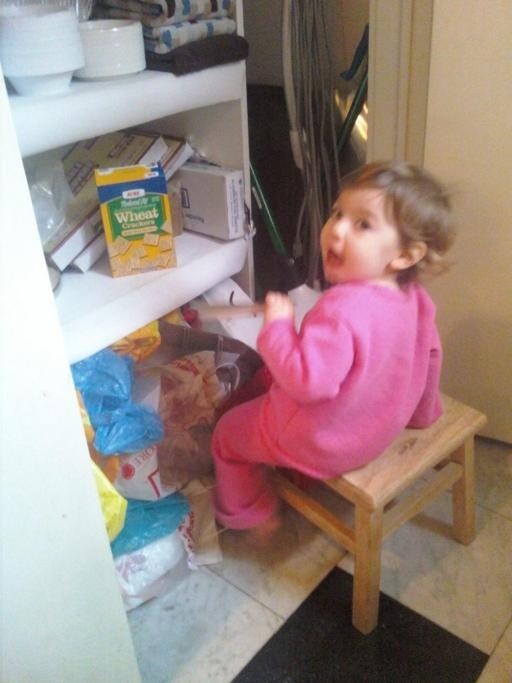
[211,159,459,546]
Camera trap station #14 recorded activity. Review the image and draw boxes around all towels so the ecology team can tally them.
[92,0,231,25]
[141,19,236,52]
[146,35,248,76]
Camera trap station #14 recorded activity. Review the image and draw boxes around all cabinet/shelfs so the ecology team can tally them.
[0,0,255,610]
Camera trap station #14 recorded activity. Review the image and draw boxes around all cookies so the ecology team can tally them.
[107,220,172,277]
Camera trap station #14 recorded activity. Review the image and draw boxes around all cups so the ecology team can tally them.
[78,18,148,81]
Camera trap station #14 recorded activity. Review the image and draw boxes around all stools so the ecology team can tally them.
[261,391,489,634]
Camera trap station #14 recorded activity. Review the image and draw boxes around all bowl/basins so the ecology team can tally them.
[6,8,84,98]
